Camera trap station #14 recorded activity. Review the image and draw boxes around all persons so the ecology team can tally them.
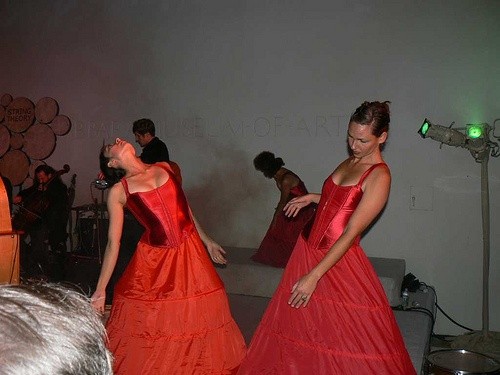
[125,120,169,257]
[282,101,391,375]
[12,165,68,257]
[91,137,227,375]
[0,281,115,375]
[254,151,314,269]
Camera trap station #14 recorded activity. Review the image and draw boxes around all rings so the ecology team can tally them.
[302,296,307,301]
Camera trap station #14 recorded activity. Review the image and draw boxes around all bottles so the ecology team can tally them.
[401,288,409,309]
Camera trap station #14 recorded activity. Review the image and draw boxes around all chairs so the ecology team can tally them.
[66,174,77,255]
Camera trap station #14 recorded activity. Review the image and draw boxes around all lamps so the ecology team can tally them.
[466,125,487,152]
[417,118,464,147]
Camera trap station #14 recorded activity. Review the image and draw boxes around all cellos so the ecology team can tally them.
[12,163,70,239]
